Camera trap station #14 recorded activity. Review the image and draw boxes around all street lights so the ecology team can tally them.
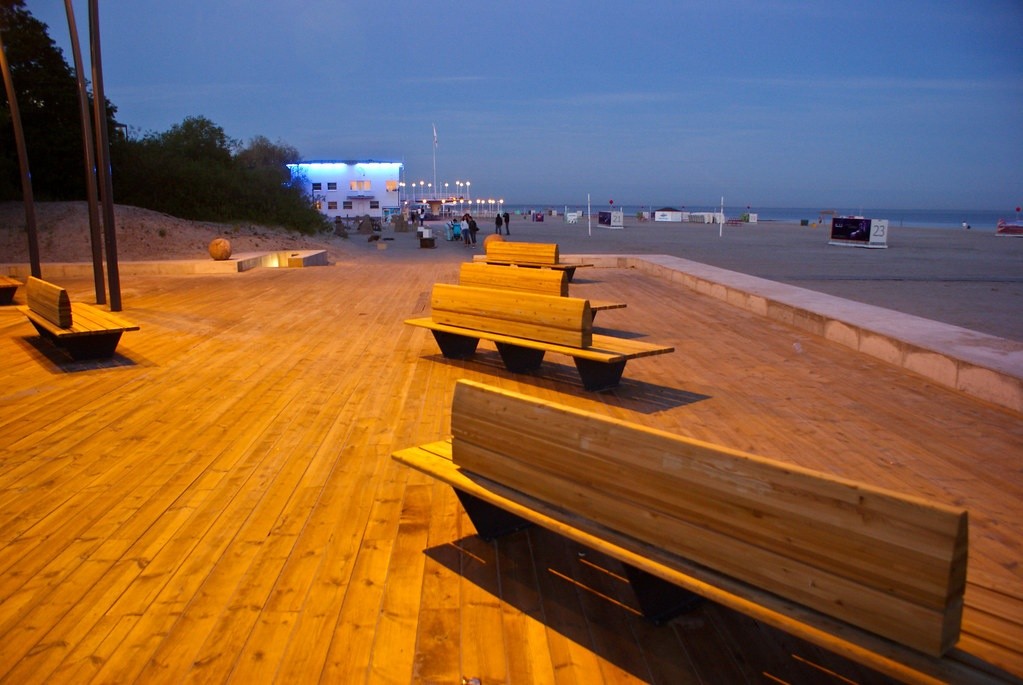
[399,180,504,219]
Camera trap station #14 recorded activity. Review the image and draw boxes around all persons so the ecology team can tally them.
[495,213,503,235]
[411,213,415,224]
[503,212,510,235]
[450,218,463,241]
[458,213,478,248]
[962,221,968,233]
[419,212,424,226]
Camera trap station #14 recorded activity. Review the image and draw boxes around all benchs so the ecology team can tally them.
[459,264,627,322]
[0,274,24,304]
[474,241,594,280]
[394,377,1022,684]
[408,283,674,390]
[17,277,140,362]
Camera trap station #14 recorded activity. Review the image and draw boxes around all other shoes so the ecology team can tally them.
[467,240,471,244]
[472,245,475,248]
[464,240,467,244]
